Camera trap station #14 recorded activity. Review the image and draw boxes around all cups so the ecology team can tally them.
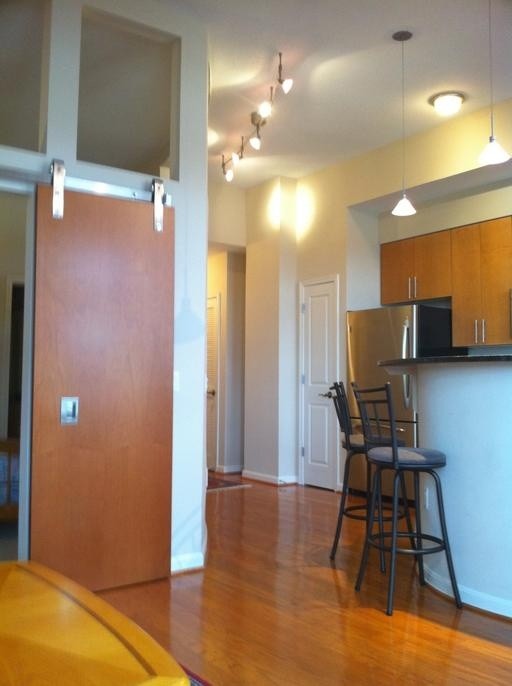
[474,318,486,345]
[407,274,417,299]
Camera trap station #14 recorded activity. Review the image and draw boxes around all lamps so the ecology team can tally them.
[426,91,466,116]
[477,0,512,168]
[220,51,294,181]
[390,29,418,219]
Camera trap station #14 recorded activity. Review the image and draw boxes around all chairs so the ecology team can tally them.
[351,379,462,616]
[328,380,417,573]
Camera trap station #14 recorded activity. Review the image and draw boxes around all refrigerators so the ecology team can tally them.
[345,303,451,505]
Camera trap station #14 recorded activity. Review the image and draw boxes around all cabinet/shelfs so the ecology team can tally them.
[379,227,451,305]
[452,216,511,349]
